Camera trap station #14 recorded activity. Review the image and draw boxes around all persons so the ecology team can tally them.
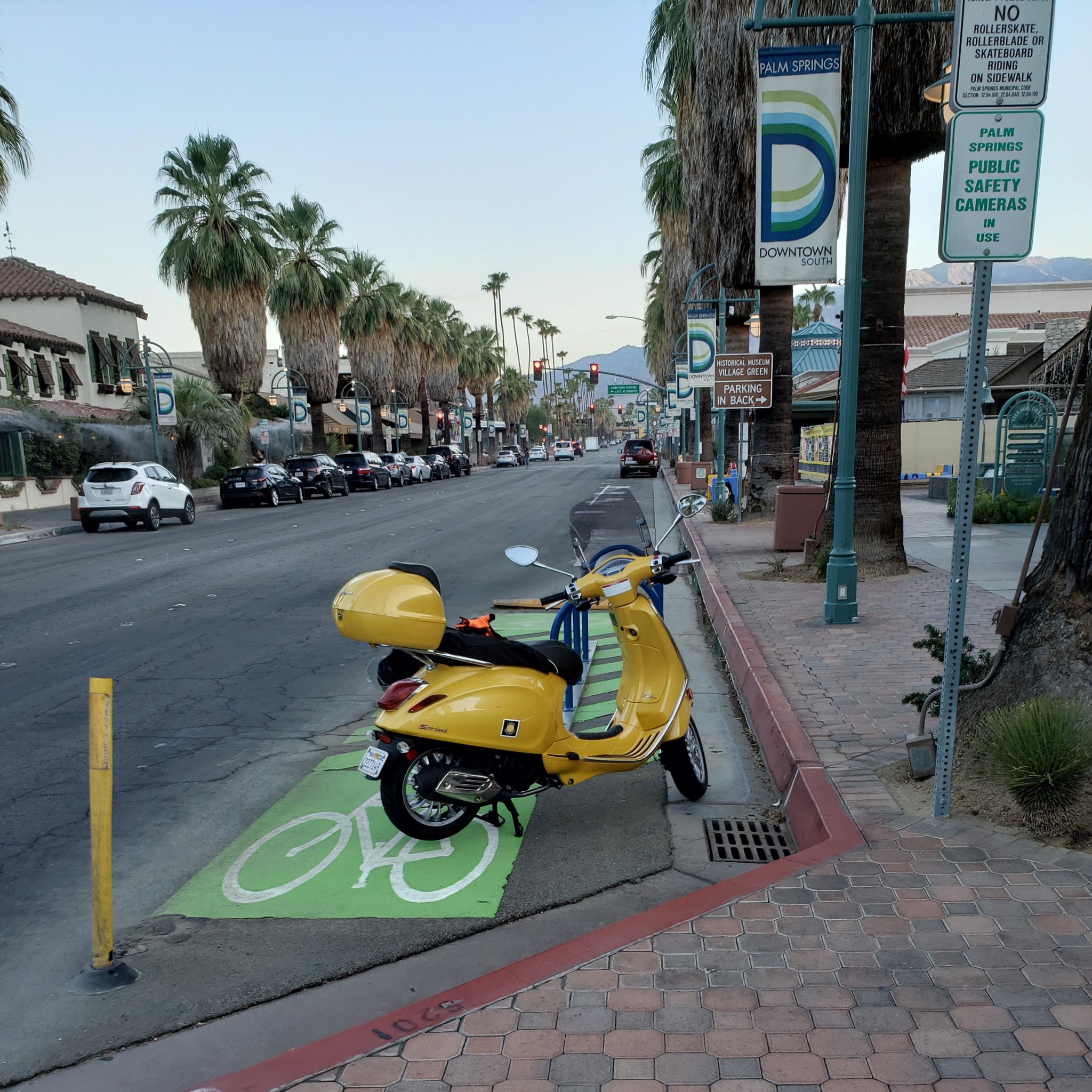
[523,446,530,469]
[399,448,408,458]
[251,449,266,464]
[280,462,285,468]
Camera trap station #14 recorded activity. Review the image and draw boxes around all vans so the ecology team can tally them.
[553,441,576,461]
[427,445,471,478]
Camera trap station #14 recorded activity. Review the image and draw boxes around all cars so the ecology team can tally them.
[529,446,549,461]
[496,450,518,467]
[220,464,306,508]
[610,436,627,445]
[599,443,607,448]
[378,452,451,487]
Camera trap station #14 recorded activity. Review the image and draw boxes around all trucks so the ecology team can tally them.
[585,437,599,451]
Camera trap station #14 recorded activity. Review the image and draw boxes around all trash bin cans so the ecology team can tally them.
[691,462,713,490]
[775,484,826,551]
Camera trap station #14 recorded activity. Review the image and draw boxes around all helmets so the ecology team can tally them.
[377,649,429,693]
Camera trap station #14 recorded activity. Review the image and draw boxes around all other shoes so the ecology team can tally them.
[526,466,530,468]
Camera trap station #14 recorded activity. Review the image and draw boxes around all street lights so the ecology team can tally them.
[381,387,409,454]
[636,388,661,440]
[680,261,762,510]
[646,386,651,438]
[449,389,473,453]
[116,336,173,467]
[481,406,495,466]
[268,366,309,455]
[339,378,372,452]
[739,0,962,626]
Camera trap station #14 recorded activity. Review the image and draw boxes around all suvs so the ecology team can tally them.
[333,450,392,491]
[284,453,350,499]
[78,461,196,533]
[572,441,584,457]
[502,445,525,466]
[617,438,661,479]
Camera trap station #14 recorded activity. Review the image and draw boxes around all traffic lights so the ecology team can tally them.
[618,404,622,414]
[533,361,542,381]
[590,364,599,384]
[590,404,594,413]
[437,413,444,430]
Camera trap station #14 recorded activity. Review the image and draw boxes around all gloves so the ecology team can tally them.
[457,613,516,643]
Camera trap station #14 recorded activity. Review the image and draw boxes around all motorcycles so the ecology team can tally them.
[332,491,712,841]
